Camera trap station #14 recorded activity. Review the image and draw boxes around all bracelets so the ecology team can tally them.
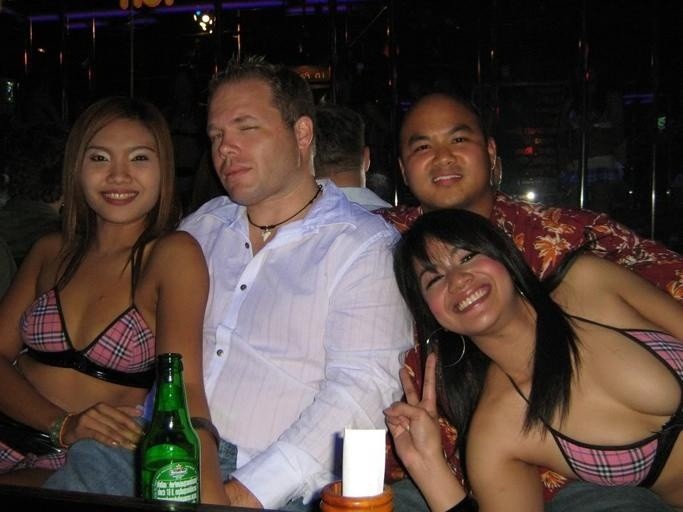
[49,415,61,448]
[59,413,75,448]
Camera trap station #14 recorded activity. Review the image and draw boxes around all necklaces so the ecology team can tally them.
[246,184,324,242]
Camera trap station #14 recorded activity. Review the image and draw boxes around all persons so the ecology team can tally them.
[314,104,393,212]
[382,208,683,512]
[174,52,415,509]
[0,95,231,506]
[370,91,683,305]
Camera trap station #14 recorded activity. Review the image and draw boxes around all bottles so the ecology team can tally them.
[138,353,200,506]
